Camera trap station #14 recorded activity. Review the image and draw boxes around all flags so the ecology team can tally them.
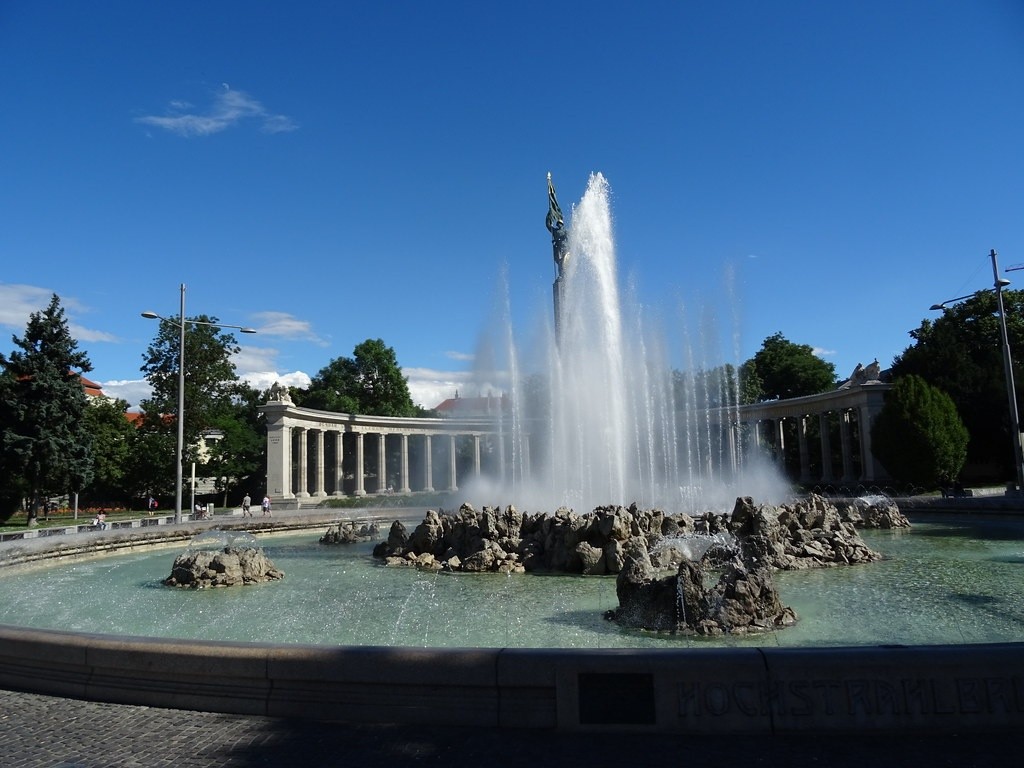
[549,183,563,222]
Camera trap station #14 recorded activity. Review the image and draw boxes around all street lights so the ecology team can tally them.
[141,283,258,524]
[928,247,1024,511]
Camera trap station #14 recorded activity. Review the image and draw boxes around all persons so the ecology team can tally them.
[546,211,569,279]
[96,509,106,531]
[241,493,253,518]
[262,495,273,518]
[195,501,212,520]
[148,495,155,516]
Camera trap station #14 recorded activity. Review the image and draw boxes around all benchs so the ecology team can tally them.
[947,490,973,497]
[0,513,200,543]
